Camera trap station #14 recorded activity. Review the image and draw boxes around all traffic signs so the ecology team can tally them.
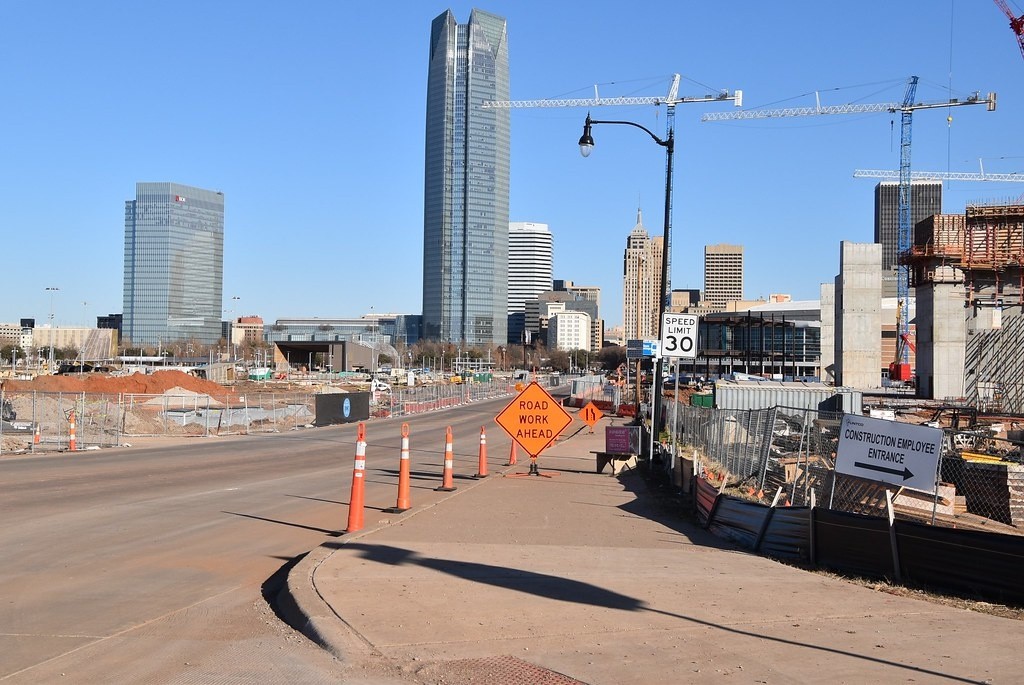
[832,414,944,492]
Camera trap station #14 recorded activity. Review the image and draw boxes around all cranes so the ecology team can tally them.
[482,74,742,311]
[852,158,1024,183]
[700,75,998,382]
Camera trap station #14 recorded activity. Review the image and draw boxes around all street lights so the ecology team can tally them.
[578,103,676,460]
